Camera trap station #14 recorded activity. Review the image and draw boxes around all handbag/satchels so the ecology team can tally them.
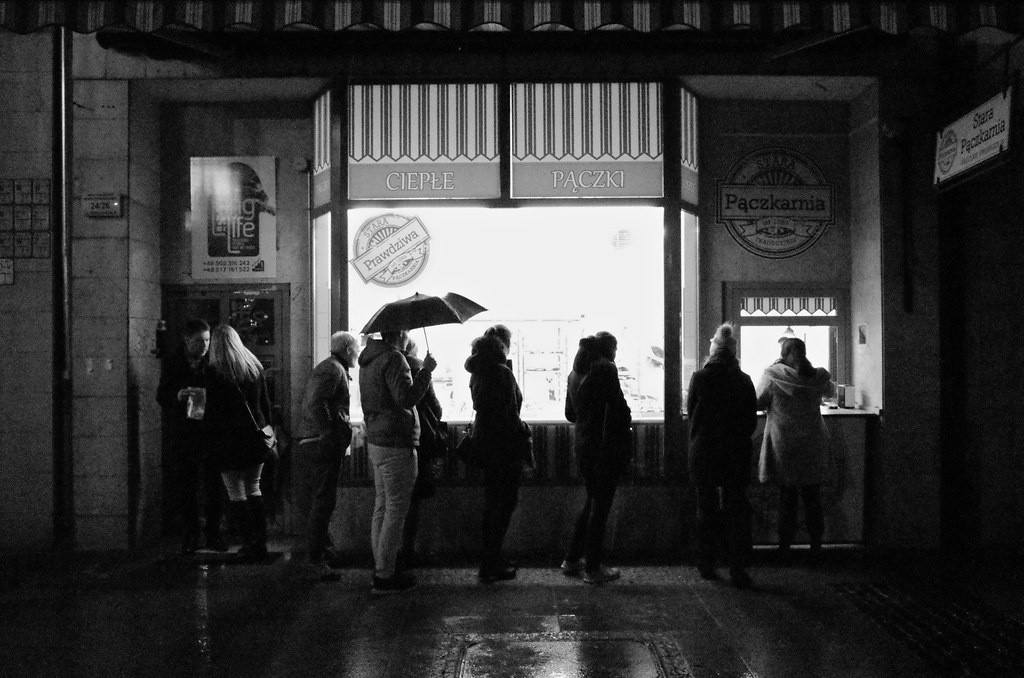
[251,425,283,461]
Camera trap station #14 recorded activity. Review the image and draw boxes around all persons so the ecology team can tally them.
[465,328,532,582]
[559,332,631,583]
[160,319,275,560]
[754,338,837,570]
[686,325,758,580]
[359,329,441,596]
[297,330,355,568]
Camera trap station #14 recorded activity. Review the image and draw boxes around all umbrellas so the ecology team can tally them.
[360,292,487,354]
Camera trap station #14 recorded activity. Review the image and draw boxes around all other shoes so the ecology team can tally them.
[560,559,588,576]
[582,565,622,586]
[369,572,422,595]
[221,542,272,566]
[805,545,826,563]
[771,543,793,561]
[476,558,522,585]
[698,557,720,582]
[727,565,750,585]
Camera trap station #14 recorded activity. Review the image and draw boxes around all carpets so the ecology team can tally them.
[822,576,1024,678]
